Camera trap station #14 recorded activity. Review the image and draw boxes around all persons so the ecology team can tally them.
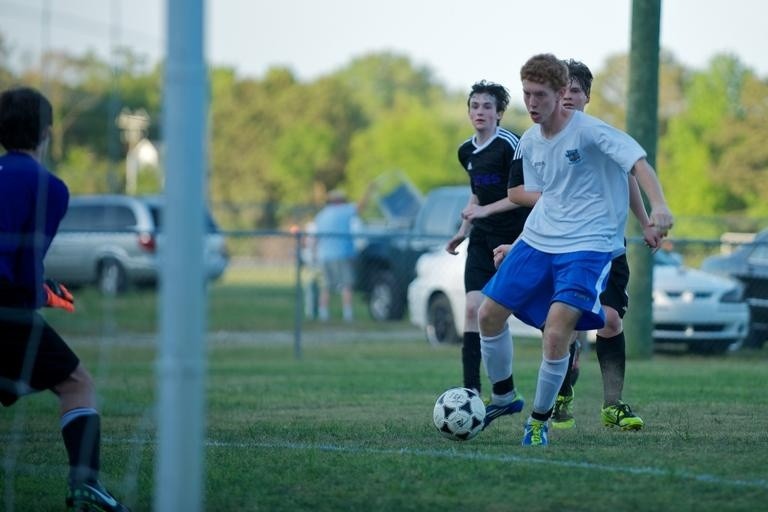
[305,189,371,318]
[479,55,674,448]
[492,59,661,430]
[444,79,533,395]
[0,86,123,511]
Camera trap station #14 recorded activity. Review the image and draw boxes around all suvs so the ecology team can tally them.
[42,191,227,290]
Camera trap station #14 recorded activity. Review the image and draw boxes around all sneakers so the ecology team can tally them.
[551,385,575,430]
[62,476,124,511]
[318,312,355,324]
[521,415,551,449]
[481,392,525,431]
[600,400,644,432]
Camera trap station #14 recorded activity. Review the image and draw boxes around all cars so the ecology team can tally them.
[703,226,766,349]
[407,230,748,357]
[351,177,475,323]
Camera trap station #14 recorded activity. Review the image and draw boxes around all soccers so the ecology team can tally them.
[433,388,486,440]
